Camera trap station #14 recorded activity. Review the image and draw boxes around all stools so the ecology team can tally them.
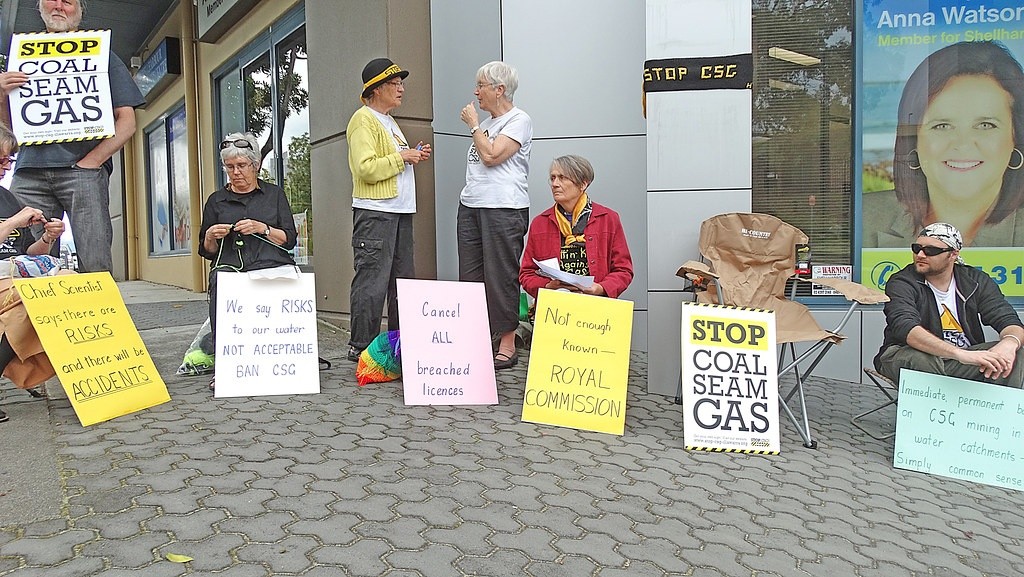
[850,366,898,442]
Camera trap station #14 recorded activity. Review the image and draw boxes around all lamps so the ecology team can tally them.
[130,48,149,69]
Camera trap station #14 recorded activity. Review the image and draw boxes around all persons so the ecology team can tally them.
[0,124,80,421]
[346,58,431,360]
[520,155,634,299]
[198,132,298,389]
[863,41,1024,248]
[874,223,1024,389]
[1,0,147,276]
[456,62,532,369]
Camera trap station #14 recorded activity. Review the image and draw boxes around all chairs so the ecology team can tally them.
[674,212,890,450]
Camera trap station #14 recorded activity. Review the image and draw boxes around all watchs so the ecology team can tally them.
[470,126,479,134]
[262,224,270,238]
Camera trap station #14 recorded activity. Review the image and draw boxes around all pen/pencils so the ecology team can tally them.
[408,145,422,165]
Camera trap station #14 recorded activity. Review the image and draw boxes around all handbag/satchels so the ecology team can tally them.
[355,329,401,385]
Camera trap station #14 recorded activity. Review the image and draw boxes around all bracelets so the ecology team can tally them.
[1003,335,1021,350]
[42,234,54,244]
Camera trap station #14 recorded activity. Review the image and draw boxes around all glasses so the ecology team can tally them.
[219,139,253,156]
[385,81,404,88]
[0,158,13,165]
[475,83,493,90]
[912,244,954,256]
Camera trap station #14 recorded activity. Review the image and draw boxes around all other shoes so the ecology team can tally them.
[348,348,363,362]
[494,351,518,369]
[209,374,215,390]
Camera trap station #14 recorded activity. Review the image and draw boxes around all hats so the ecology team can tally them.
[360,58,410,105]
